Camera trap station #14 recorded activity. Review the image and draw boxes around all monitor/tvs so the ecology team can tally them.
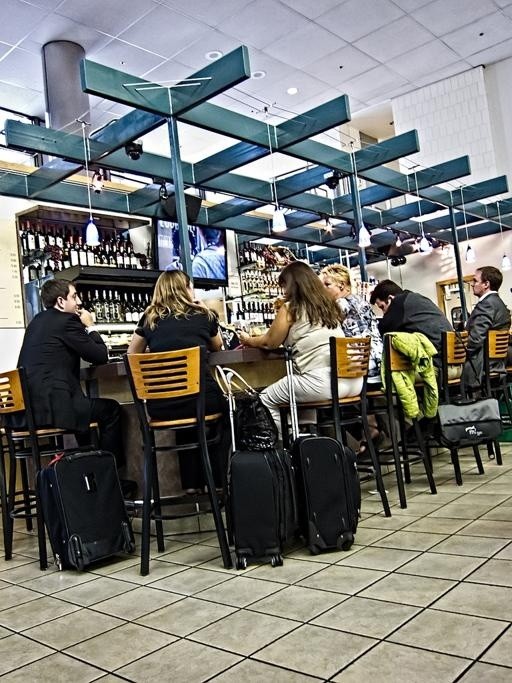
[155,220,228,291]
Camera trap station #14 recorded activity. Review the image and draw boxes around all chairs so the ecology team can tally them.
[0,368,98,572]
[124,348,234,575]
[485,330,511,464]
[279,337,391,517]
[367,331,437,507]
[403,331,484,484]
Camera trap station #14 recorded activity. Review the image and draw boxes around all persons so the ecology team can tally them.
[369,280,465,448]
[318,263,385,462]
[238,262,364,441]
[167,225,200,269]
[458,266,512,403]
[18,278,134,498]
[191,227,225,279]
[126,269,224,493]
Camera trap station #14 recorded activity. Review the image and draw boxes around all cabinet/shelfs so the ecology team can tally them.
[436,275,479,329]
[15,203,158,359]
[223,232,311,336]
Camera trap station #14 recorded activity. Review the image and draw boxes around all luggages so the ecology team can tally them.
[227,374,298,570]
[286,346,361,556]
[35,445,136,573]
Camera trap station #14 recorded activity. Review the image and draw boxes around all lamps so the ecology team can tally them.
[324,217,334,233]
[496,201,511,272]
[81,124,100,246]
[325,169,339,188]
[460,187,476,262]
[92,173,104,195]
[389,235,414,266]
[351,149,372,248]
[159,181,169,201]
[273,179,288,233]
[123,140,144,161]
[414,172,432,254]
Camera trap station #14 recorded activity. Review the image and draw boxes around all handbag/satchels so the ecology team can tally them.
[437,332,502,450]
[214,364,279,453]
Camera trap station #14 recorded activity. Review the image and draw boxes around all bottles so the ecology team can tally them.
[28,259,37,283]
[240,238,244,266]
[69,236,79,266]
[79,292,85,314]
[79,236,88,266]
[114,239,124,268]
[36,224,46,252]
[243,241,249,263]
[23,220,36,253]
[124,291,133,323]
[226,301,275,324]
[298,249,303,260]
[108,290,115,321]
[240,268,282,295]
[145,292,152,305]
[126,232,134,254]
[95,289,103,320]
[302,249,307,261]
[64,250,70,269]
[137,293,144,321]
[110,231,120,251]
[114,291,123,323]
[103,290,112,322]
[119,233,127,255]
[131,293,138,323]
[102,235,108,252]
[86,289,95,322]
[86,244,96,265]
[44,255,54,279]
[131,245,139,268]
[56,226,65,249]
[46,225,57,251]
[122,239,130,268]
[249,241,257,263]
[259,244,266,264]
